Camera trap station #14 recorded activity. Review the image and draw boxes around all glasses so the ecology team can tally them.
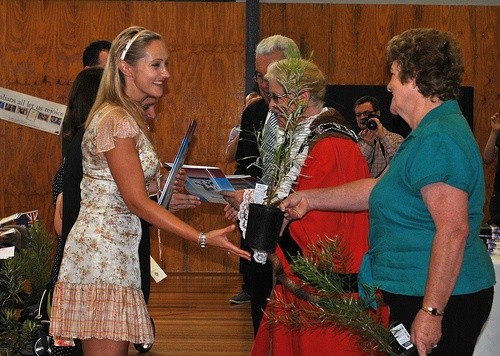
[253,73,264,84]
[266,90,292,102]
[354,109,378,117]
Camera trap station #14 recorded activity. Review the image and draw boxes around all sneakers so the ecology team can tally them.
[229,292,251,303]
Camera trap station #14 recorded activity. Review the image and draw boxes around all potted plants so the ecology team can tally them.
[243,43,319,254]
[0,220,56,356]
[261,236,420,355]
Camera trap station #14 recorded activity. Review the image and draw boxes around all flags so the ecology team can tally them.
[16,210,40,244]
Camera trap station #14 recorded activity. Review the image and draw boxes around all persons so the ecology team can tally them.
[50,40,201,304]
[219,58,390,355]
[225,35,301,340]
[276,28,495,355]
[48,26,252,355]
[353,96,405,179]
[483,113,499,225]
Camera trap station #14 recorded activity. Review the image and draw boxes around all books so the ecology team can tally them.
[479,225,500,254]
[158,119,198,210]
[183,169,256,205]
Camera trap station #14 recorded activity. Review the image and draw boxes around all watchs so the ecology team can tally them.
[421,305,444,316]
[200,232,206,248]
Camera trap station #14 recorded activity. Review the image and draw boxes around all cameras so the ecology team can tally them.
[367,114,381,130]
[388,321,417,356]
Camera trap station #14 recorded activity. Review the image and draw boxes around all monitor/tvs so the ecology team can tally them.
[324,84,474,148]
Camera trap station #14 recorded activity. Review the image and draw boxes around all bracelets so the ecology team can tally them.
[197,232,203,247]
